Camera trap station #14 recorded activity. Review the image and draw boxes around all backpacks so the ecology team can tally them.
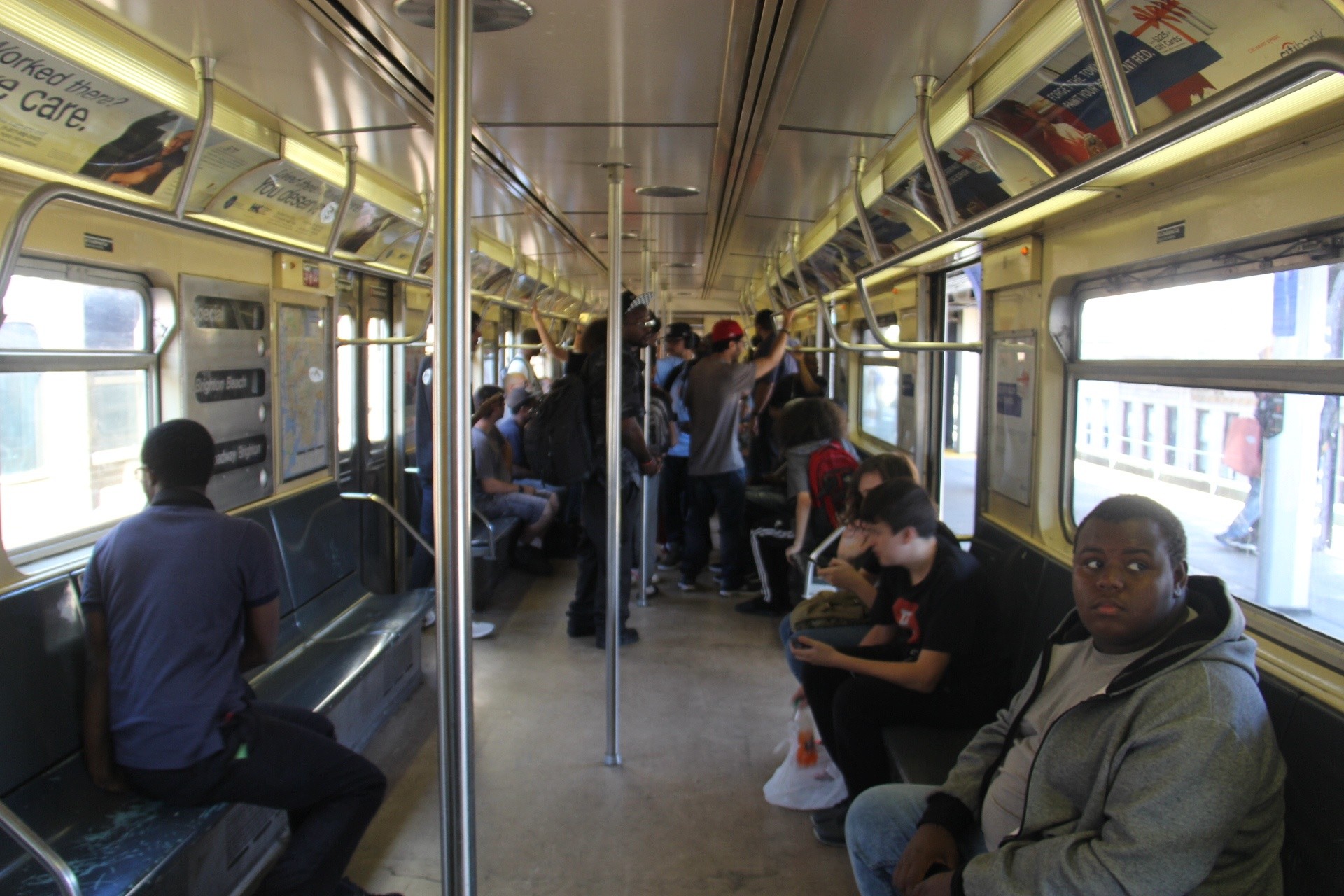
[524,355,606,485]
[809,439,859,530]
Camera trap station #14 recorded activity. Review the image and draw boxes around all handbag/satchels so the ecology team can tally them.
[790,590,870,632]
[762,722,848,810]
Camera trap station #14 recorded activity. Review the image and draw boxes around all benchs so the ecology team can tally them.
[1,465,526,896]
[791,443,1344,895]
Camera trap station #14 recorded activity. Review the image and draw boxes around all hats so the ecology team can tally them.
[712,319,744,342]
[472,385,503,417]
[665,322,692,340]
[506,388,542,409]
[621,290,654,314]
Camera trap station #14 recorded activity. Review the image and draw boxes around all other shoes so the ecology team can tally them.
[568,619,639,649]
[735,594,782,616]
[422,611,436,627]
[809,807,849,847]
[632,541,762,599]
[472,619,496,639]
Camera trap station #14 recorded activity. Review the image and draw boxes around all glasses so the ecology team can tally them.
[134,467,159,483]
[626,319,657,330]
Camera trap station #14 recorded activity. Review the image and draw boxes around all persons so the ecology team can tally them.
[82,419,387,896]
[778,451,1002,847]
[409,290,858,647]
[843,496,1285,896]
[1216,382,1262,552]
